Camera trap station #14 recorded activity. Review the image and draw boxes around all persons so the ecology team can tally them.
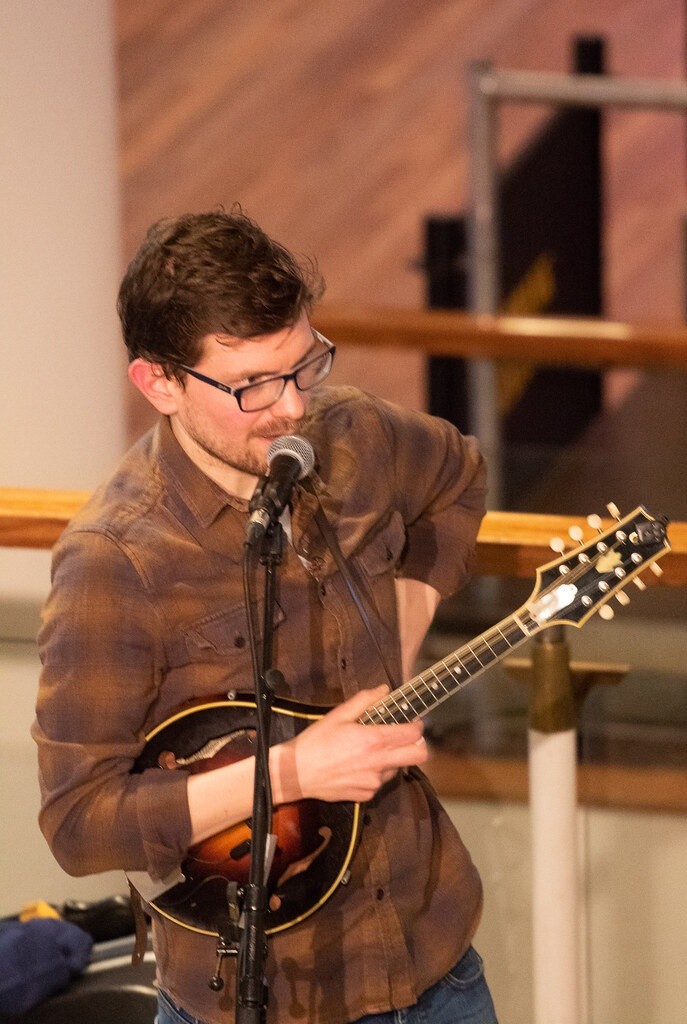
[28,200,498,1024]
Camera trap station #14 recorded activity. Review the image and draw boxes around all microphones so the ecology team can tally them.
[242,434,314,548]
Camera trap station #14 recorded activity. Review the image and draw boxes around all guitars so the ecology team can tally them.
[118,492,676,945]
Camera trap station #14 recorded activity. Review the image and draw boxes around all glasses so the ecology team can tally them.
[171,327,337,414]
